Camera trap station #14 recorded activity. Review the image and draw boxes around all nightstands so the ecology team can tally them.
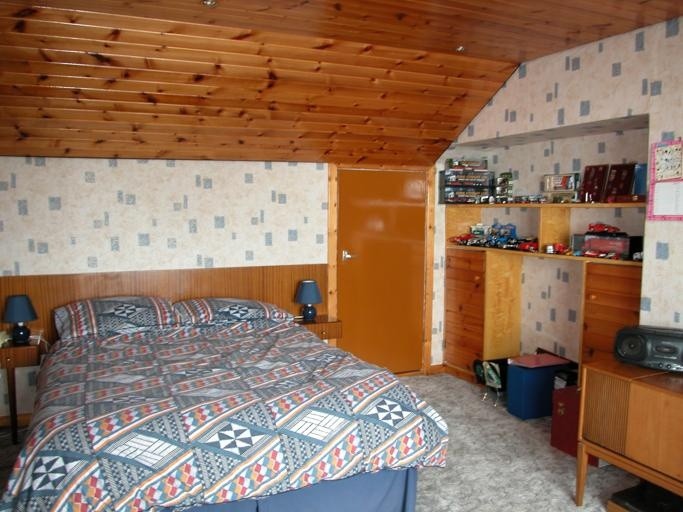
[297,316,342,339]
[0,329,45,444]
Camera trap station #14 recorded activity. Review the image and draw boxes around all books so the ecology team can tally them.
[576,163,609,203]
[601,162,638,204]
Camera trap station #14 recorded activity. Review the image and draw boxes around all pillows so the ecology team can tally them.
[53,296,173,340]
[173,298,291,323]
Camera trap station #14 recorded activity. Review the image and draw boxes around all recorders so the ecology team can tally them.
[613,324,683,373]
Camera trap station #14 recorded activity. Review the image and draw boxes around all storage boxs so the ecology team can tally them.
[551,387,611,468]
[506,362,572,420]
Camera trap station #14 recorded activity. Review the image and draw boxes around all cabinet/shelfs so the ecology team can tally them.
[573,359,683,505]
[445,203,539,385]
[539,203,642,388]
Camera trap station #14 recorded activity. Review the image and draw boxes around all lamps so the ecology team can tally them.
[294,280,322,323]
[1,295,36,344]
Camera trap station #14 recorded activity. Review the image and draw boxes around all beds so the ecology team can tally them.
[0,296,447,512]
